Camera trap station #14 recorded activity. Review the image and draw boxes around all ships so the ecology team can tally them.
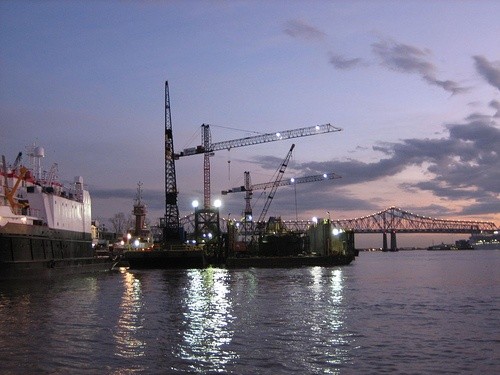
[1,142,115,278]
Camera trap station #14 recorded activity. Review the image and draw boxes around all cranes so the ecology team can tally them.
[180,121,345,237]
